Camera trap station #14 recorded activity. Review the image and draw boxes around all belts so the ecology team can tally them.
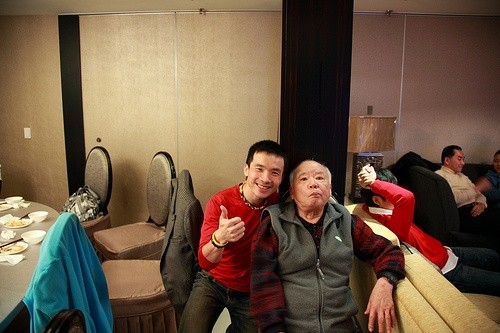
[457,202,478,211]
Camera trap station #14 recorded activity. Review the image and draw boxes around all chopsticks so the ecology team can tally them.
[0,238,23,248]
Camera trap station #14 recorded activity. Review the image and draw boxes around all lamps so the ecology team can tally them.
[345,106,397,200]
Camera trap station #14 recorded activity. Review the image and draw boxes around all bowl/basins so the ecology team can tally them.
[21,230,46,244]
[28,211,48,222]
[5,196,23,204]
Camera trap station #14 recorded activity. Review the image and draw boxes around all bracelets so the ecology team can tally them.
[212,233,228,248]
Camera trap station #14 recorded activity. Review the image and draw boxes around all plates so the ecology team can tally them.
[3,219,33,228]
[0,198,6,204]
[0,241,28,255]
[0,204,11,211]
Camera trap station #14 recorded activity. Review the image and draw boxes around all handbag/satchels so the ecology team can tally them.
[59,185,100,222]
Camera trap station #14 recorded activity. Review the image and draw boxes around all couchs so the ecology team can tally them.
[344,202,500,333]
[386,152,500,245]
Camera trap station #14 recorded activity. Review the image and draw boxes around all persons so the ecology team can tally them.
[177,140,285,333]
[358,164,500,297]
[250,160,406,333]
[474,150,500,201]
[434,145,500,253]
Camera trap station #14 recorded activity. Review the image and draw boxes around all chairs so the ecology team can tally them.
[94,150,175,259]
[22,211,114,332]
[61,146,114,252]
[99,170,204,333]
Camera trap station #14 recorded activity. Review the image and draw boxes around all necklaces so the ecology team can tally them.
[240,182,268,210]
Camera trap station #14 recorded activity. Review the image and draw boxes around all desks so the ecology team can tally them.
[0,199,60,333]
